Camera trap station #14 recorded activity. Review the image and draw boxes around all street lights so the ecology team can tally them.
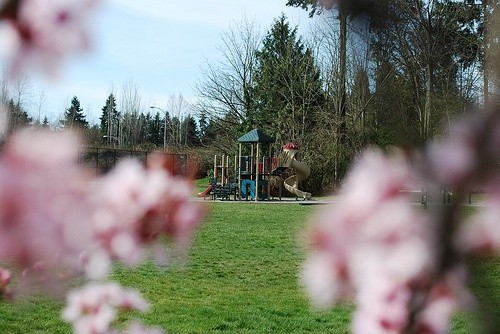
[103,135,120,146]
[150,106,167,149]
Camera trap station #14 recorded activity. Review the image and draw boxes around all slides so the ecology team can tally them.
[197,184,212,197]
[278,144,312,201]
